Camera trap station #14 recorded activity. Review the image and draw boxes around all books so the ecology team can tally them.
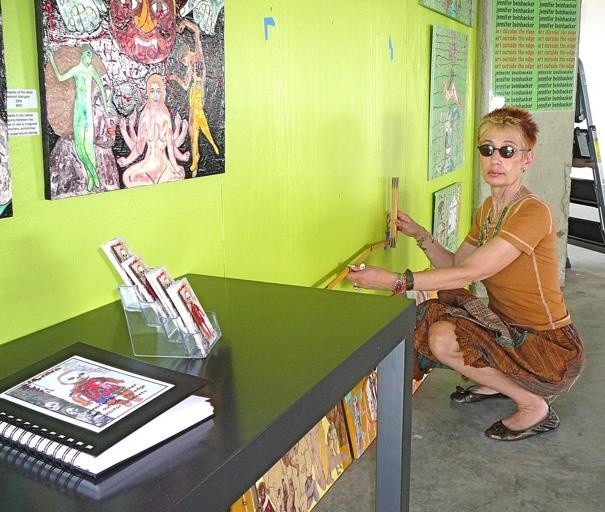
[99,238,220,356]
[0,340,216,482]
[0,419,216,502]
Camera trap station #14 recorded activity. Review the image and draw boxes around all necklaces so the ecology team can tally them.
[468,184,527,296]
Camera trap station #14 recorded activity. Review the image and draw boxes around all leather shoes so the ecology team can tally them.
[484,405,559,441]
[451,384,510,404]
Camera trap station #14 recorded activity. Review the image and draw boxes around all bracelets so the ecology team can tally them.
[416,231,435,250]
[393,268,414,295]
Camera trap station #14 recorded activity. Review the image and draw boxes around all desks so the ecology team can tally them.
[0,272,416,512]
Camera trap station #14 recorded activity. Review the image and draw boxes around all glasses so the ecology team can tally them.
[477,144,532,158]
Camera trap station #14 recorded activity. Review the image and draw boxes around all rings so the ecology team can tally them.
[352,279,361,292]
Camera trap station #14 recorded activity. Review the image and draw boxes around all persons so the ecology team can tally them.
[348,107,586,439]
